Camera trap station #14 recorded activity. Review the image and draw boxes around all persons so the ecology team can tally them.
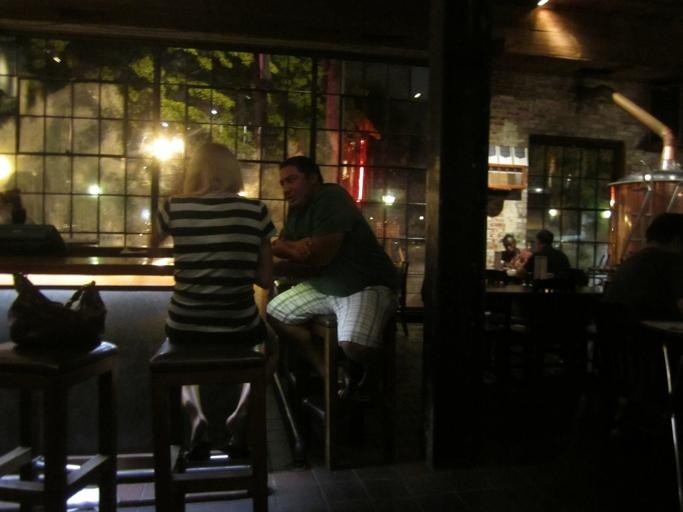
[267,156,400,398]
[600,214,682,323]
[0,192,38,225]
[498,230,572,282]
[151,142,276,468]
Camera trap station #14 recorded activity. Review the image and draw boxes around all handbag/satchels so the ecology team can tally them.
[8,270,107,354]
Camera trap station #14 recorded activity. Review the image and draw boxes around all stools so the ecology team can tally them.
[290,312,402,472]
[1,340,119,511]
[148,336,277,512]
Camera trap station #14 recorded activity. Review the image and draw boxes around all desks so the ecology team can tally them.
[485,285,603,412]
